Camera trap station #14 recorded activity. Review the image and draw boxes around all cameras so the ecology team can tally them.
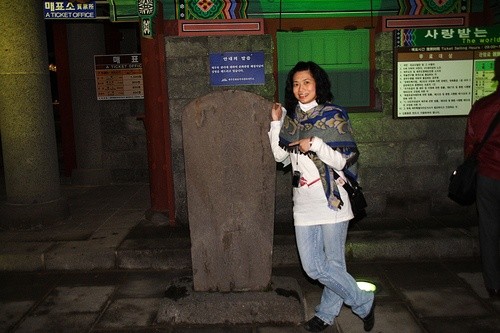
[292,171,301,187]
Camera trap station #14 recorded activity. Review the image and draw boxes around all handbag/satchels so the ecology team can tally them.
[448,163,476,204]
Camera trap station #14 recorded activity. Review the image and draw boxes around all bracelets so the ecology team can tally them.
[310,137,313,147]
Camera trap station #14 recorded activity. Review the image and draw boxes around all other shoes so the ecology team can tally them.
[364,292,377,331]
[305,315,329,332]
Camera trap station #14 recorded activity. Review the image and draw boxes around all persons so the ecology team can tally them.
[464,56,500,302]
[268,61,377,333]
